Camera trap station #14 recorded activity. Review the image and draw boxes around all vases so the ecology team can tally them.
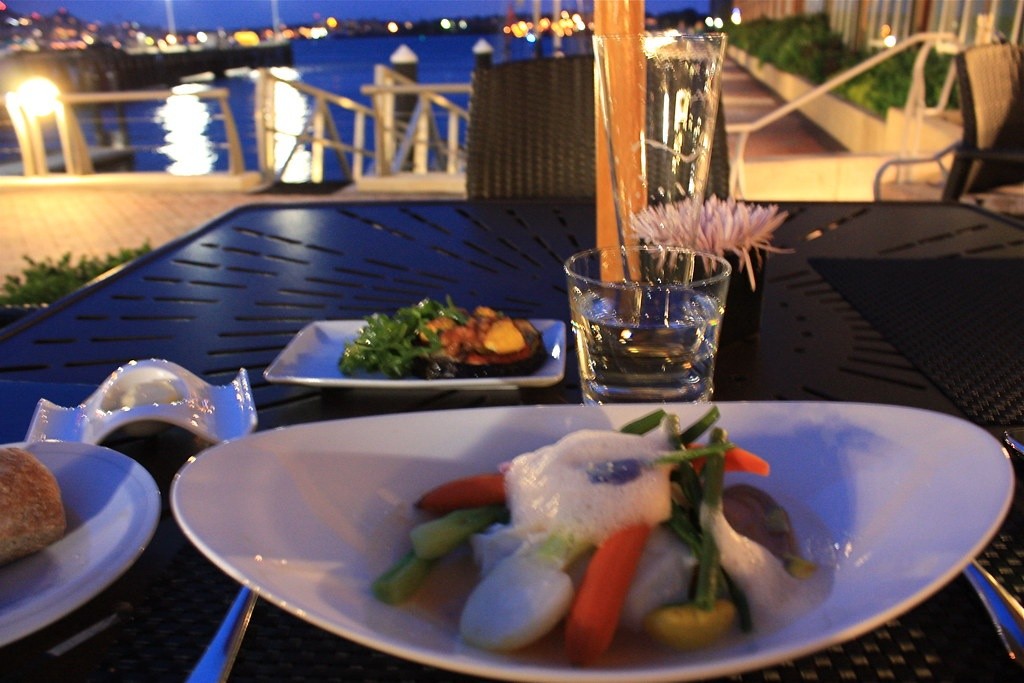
[639,242,768,347]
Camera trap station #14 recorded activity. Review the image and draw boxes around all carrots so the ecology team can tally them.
[563,521,649,667]
[415,473,509,510]
[680,441,770,477]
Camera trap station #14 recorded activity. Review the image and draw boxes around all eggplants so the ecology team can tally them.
[416,318,545,378]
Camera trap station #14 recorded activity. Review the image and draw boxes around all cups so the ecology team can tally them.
[589,27,729,293]
[561,247,733,405]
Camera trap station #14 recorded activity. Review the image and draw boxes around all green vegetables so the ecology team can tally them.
[337,295,466,377]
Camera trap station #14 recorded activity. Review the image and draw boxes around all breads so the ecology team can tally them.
[0,443,70,568]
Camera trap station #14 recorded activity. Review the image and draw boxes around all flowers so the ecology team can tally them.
[627,194,795,292]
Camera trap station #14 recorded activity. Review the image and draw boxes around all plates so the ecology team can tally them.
[1,441,163,648]
[264,315,569,394]
[170,398,1017,682]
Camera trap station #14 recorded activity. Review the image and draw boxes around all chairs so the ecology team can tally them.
[942,42,1024,217]
[464,52,730,202]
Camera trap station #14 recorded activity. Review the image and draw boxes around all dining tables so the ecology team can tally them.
[0,202,1024,683]
